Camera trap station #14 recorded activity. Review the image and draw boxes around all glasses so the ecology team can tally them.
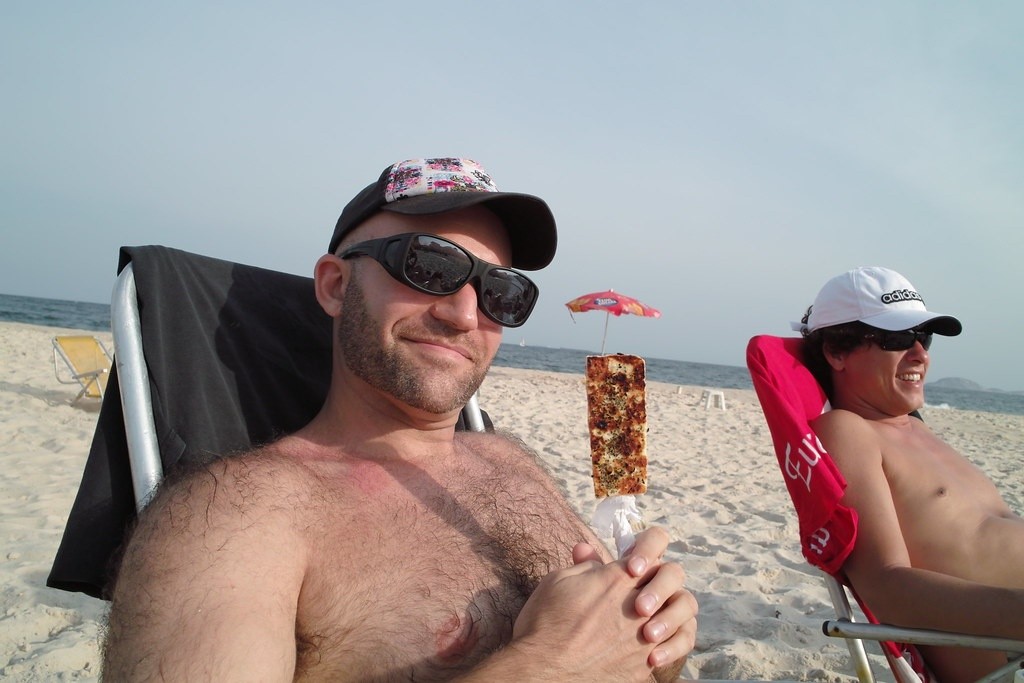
[338,232,539,329]
[849,328,932,351]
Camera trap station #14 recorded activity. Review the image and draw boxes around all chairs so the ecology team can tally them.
[745,335,1024,683]
[45,247,495,605]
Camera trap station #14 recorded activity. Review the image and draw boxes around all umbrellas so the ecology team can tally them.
[565,289,661,356]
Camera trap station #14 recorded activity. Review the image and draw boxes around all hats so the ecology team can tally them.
[807,266,963,336]
[328,157,557,271]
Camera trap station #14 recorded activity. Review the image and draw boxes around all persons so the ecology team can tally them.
[791,264,1024,683]
[101,156,700,683]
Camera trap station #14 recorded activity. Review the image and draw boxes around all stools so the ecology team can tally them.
[699,389,726,411]
